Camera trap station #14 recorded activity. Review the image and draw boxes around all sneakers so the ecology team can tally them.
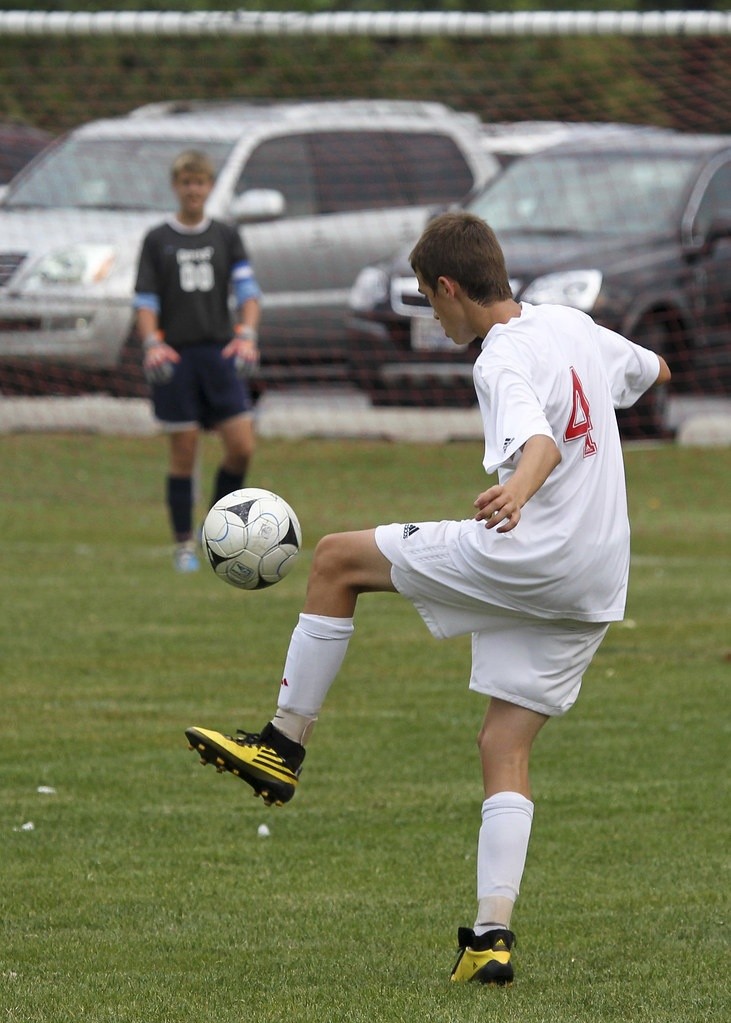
[185,726,301,807]
[450,928,517,985]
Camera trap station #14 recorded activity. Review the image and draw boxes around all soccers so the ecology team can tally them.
[199,487,304,592]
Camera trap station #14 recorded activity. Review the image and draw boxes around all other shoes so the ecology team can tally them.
[175,540,199,573]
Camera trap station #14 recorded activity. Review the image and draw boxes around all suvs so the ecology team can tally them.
[1,100,505,401]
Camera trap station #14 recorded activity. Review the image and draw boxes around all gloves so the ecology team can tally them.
[142,331,178,385]
[221,325,260,381]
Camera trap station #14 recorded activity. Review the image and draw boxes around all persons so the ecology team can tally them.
[186,212,670,985]
[131,150,264,576]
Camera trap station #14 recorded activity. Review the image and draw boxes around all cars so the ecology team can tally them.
[332,123,731,431]
[0,122,88,198]
[475,115,690,189]
